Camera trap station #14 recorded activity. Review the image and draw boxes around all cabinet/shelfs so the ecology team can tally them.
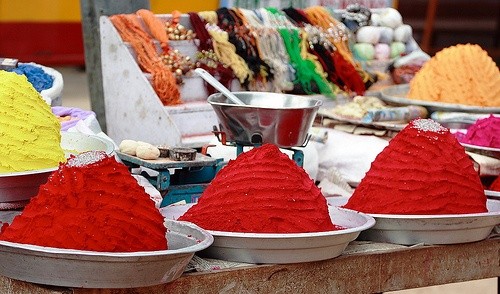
[99,8,428,150]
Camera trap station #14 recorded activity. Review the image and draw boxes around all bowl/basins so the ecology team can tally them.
[159,204,377,264]
[439,120,500,160]
[0,210,215,289]
[378,83,500,115]
[0,131,116,204]
[326,196,500,245]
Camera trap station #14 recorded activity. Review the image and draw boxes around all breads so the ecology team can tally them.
[119,140,159,160]
